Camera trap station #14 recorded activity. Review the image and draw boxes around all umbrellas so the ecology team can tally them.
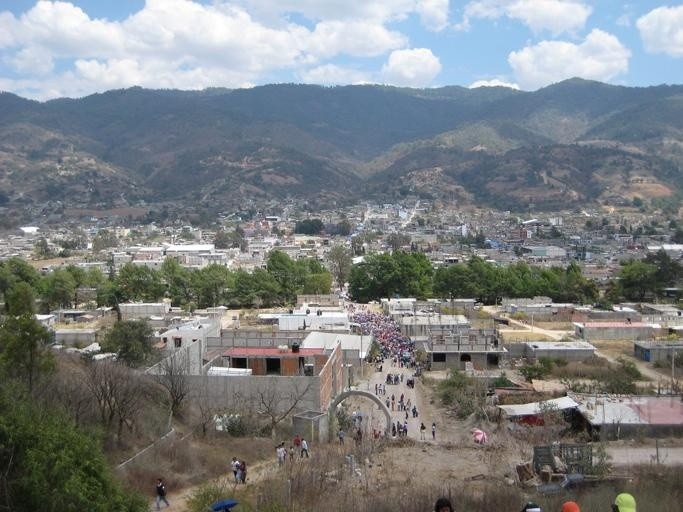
[212,499,239,511]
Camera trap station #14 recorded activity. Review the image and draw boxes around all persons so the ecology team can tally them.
[155,478,169,511]
[231,456,247,484]
[338,428,346,445]
[375,383,378,395]
[384,393,436,441]
[379,430,384,440]
[373,429,379,439]
[344,304,416,388]
[434,497,455,512]
[275,433,309,467]
[383,385,386,395]
[522,500,541,512]
[378,383,383,394]
[356,426,362,439]
